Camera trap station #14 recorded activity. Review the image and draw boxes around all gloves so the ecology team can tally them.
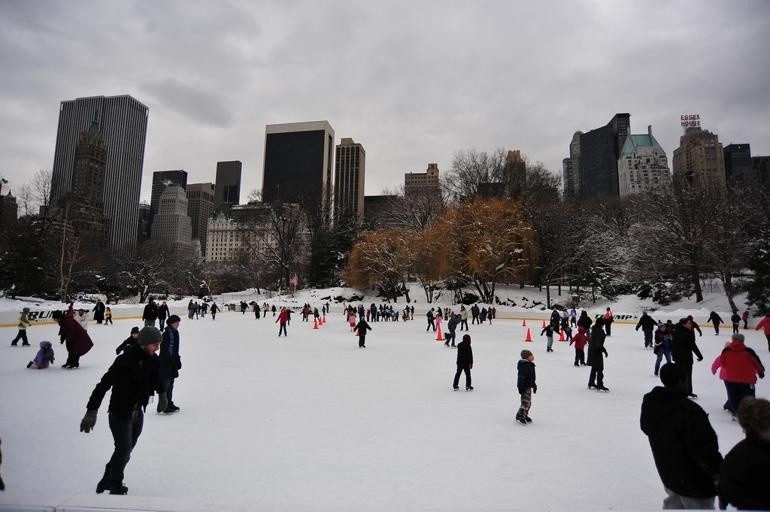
[158,393,168,413]
[80,410,97,432]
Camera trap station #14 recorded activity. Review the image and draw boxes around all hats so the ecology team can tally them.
[137,327,162,345]
[166,315,179,326]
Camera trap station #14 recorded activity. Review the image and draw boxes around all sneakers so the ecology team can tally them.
[165,402,178,412]
[588,383,608,390]
[96,480,127,494]
[516,413,532,425]
[62,362,79,368]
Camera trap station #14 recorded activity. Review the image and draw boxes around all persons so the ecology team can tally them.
[426,304,497,391]
[514,304,770,424]
[11,299,112,369]
[342,302,414,348]
[188,299,330,337]
[80,315,183,495]
[640,364,770,512]
[142,295,171,332]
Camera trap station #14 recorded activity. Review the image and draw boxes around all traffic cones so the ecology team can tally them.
[525,328,532,341]
[522,319,527,326]
[323,316,326,323]
[558,329,566,341]
[313,319,319,329]
[541,320,547,329]
[355,329,359,336]
[319,317,323,325]
[435,327,445,341]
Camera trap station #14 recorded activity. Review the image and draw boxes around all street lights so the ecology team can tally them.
[279,215,289,294]
